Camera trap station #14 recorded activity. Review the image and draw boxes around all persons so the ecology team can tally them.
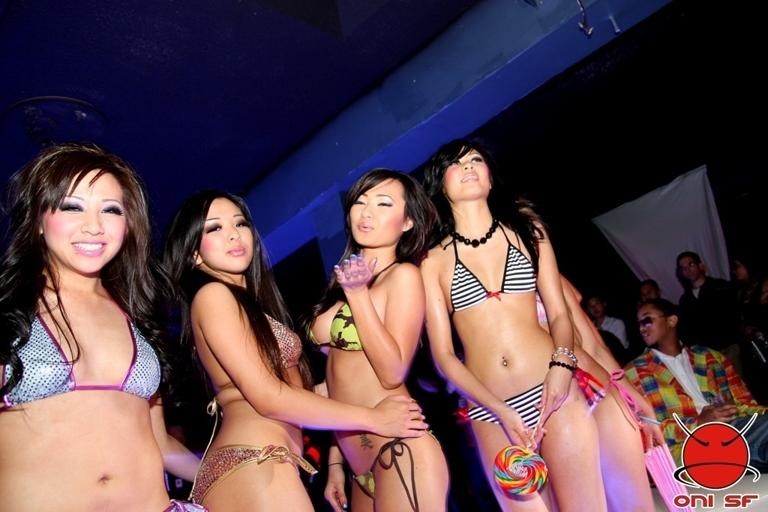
[1,142,200,512]
[158,142,768,512]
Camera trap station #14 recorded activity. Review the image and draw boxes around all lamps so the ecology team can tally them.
[576,0,593,36]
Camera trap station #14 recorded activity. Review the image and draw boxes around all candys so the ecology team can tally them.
[493,405,549,496]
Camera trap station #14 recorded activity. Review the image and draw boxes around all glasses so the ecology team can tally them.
[678,262,697,272]
[637,315,663,329]
